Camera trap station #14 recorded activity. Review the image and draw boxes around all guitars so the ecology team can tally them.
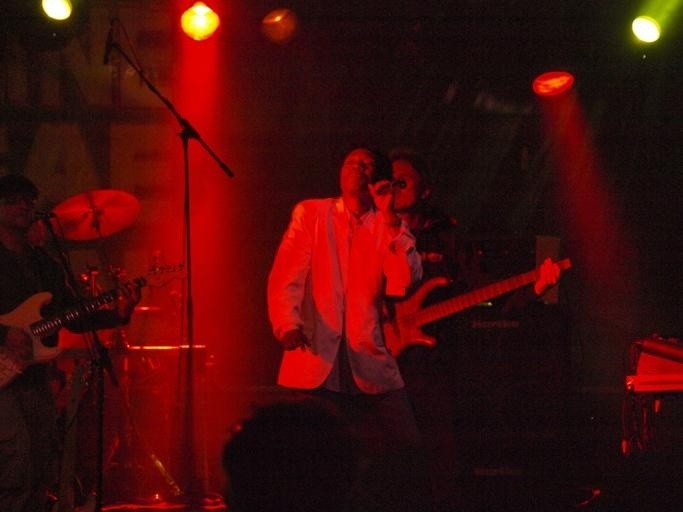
[380,256,573,357]
[0,261,184,386]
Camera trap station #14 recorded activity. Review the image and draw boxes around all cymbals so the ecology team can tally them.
[47,190,140,241]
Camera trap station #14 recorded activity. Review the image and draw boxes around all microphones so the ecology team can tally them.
[29,208,55,223]
[101,28,113,66]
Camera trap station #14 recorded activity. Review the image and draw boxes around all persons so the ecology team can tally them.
[388,151,561,503]
[264,144,424,449]
[221,394,357,512]
[0,174,144,512]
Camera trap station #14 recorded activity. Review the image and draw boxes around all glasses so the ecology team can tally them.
[1,191,38,209]
